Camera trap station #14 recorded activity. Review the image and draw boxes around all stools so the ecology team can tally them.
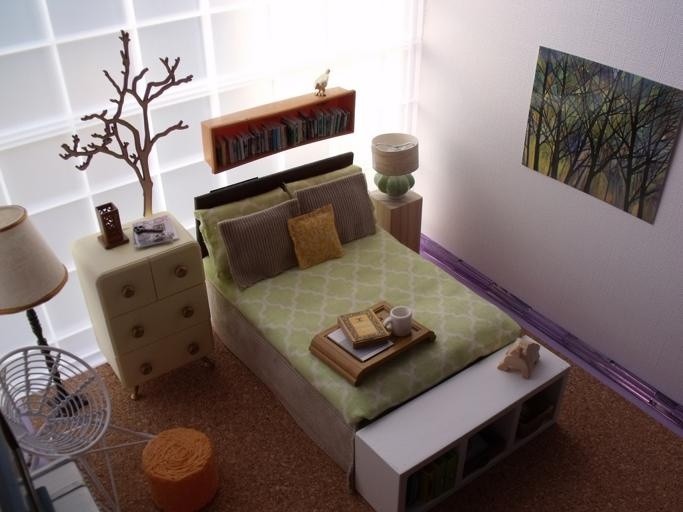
[139,428,221,512]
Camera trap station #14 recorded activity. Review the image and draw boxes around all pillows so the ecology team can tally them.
[292,171,375,248]
[218,197,297,293]
[191,184,292,287]
[284,201,345,270]
[282,164,362,198]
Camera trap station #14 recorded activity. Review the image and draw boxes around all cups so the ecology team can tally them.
[382,305,413,337]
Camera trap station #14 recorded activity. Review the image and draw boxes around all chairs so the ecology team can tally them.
[1,344,157,512]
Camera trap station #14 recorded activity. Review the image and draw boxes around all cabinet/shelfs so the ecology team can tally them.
[202,86,360,176]
[71,213,217,407]
[354,335,572,512]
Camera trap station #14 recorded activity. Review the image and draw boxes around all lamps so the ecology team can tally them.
[370,133,423,202]
[1,203,88,417]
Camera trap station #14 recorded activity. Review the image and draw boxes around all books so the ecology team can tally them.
[405,452,457,503]
[131,216,177,247]
[214,106,349,167]
[337,308,389,348]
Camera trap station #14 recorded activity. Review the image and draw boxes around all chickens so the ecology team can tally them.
[312,68,331,96]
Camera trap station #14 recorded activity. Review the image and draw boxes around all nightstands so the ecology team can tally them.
[368,192,421,255]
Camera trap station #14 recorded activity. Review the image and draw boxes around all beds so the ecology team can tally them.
[191,146,523,481]
